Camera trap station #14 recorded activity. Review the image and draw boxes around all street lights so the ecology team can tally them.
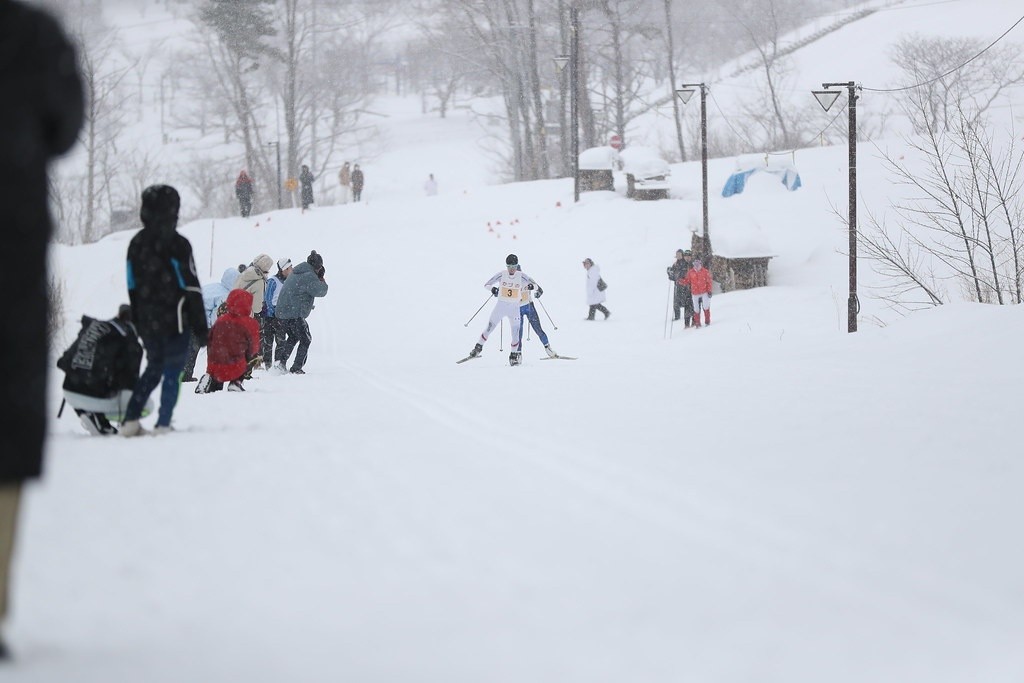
[269,141,282,208]
[675,82,708,270]
[812,82,860,332]
[553,52,576,178]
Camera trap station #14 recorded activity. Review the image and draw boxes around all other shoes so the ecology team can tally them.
[79,412,103,436]
[470,343,483,357]
[673,318,710,328]
[604,311,610,319]
[545,344,556,357]
[122,420,150,436]
[228,380,246,392]
[289,370,305,375]
[195,373,214,393]
[277,363,286,372]
[102,418,118,434]
[510,352,519,366]
[153,424,174,435]
[190,377,197,381]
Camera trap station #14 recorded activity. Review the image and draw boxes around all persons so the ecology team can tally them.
[118,185,209,437]
[516,264,558,361]
[352,164,364,201]
[182,267,241,382]
[470,254,538,365]
[339,161,350,204]
[56,304,155,436]
[236,171,253,217]
[0,0,87,657]
[582,258,610,321]
[232,254,273,379]
[667,249,712,329]
[264,258,293,370]
[300,165,315,210]
[195,289,260,394]
[275,249,328,374]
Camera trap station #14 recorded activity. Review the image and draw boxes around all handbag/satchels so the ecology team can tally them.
[597,279,607,292]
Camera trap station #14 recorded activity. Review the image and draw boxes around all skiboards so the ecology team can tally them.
[668,309,712,330]
[517,344,579,365]
[456,343,526,366]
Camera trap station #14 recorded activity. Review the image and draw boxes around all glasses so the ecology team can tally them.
[507,264,518,269]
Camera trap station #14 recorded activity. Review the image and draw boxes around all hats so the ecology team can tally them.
[505,254,518,265]
[277,258,292,271]
[307,250,323,269]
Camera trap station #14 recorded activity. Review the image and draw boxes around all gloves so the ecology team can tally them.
[491,286,498,295]
[318,266,325,278]
[528,284,534,291]
[197,331,209,347]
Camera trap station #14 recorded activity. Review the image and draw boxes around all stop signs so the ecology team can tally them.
[610,135,623,149]
